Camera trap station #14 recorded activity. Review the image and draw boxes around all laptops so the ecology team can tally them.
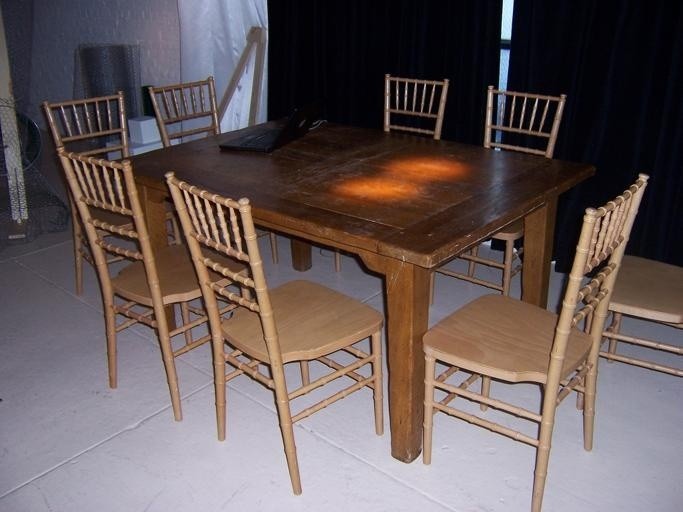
[219,95,327,154]
